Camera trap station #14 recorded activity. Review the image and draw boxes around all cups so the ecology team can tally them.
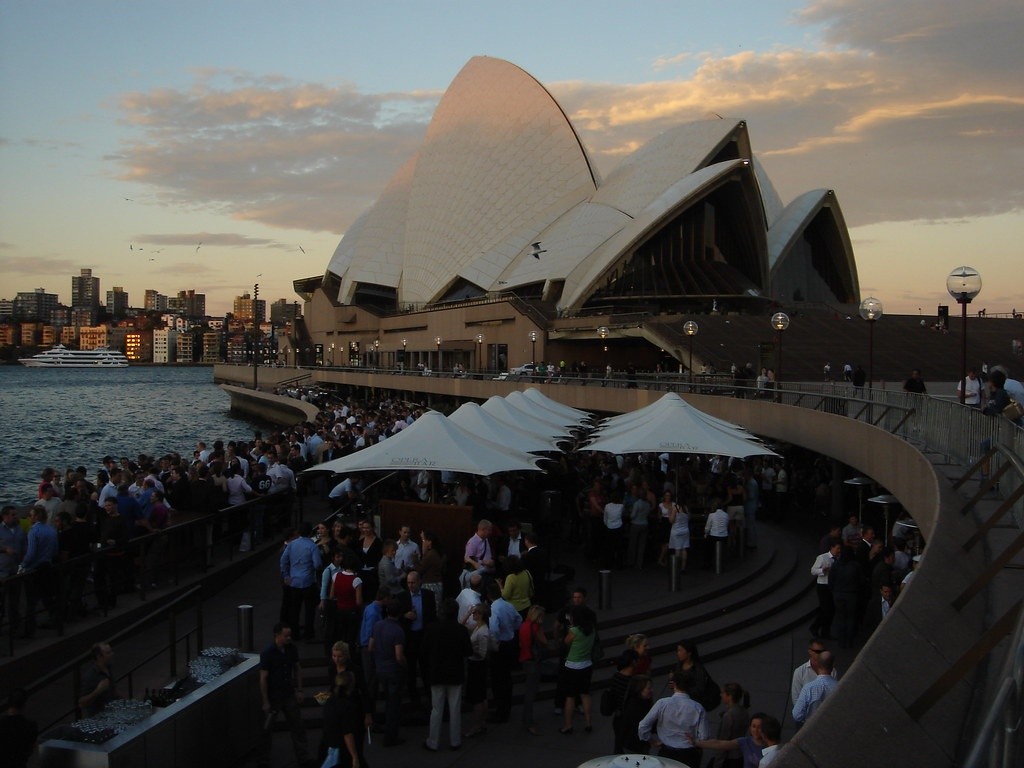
[263,713,272,729]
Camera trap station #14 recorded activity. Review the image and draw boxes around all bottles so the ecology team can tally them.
[162,691,168,708]
[142,688,150,703]
[151,688,157,706]
[157,689,162,707]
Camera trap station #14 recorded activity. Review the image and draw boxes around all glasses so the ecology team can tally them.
[809,648,826,654]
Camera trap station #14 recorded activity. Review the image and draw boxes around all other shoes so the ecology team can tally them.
[527,726,537,736]
[490,715,508,723]
[383,738,405,747]
[448,743,462,751]
[422,738,437,752]
[464,727,484,737]
[38,621,57,630]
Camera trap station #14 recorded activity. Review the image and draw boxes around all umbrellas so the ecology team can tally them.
[301,386,779,476]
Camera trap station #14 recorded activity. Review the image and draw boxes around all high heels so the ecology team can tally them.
[585,726,592,733]
[557,726,574,733]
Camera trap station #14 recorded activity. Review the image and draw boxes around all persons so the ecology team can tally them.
[453,362,465,375]
[326,359,332,367]
[701,363,707,377]
[957,368,983,408]
[605,363,612,379]
[0,421,307,640]
[979,365,1024,489]
[656,363,661,373]
[824,361,830,381]
[735,363,774,399]
[709,363,716,378]
[258,444,922,768]
[840,360,852,382]
[853,364,865,398]
[272,359,285,368]
[416,362,424,370]
[0,688,39,768]
[982,361,987,374]
[903,368,927,394]
[731,362,737,378]
[626,363,638,388]
[538,359,593,385]
[77,642,120,721]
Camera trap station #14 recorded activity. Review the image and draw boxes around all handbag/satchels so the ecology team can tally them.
[701,678,722,712]
[1001,401,1024,420]
[600,690,613,716]
[591,625,605,662]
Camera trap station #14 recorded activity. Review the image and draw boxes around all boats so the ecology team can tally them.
[16,343,129,368]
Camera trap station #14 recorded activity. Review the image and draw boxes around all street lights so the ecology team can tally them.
[374,339,380,375]
[597,326,609,386]
[529,330,539,383]
[401,338,409,376]
[770,312,790,403]
[435,335,443,378]
[859,297,883,388]
[475,333,485,378]
[946,266,982,406]
[231,342,359,368]
[682,321,699,393]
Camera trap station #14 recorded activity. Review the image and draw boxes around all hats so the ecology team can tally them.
[723,682,742,698]
